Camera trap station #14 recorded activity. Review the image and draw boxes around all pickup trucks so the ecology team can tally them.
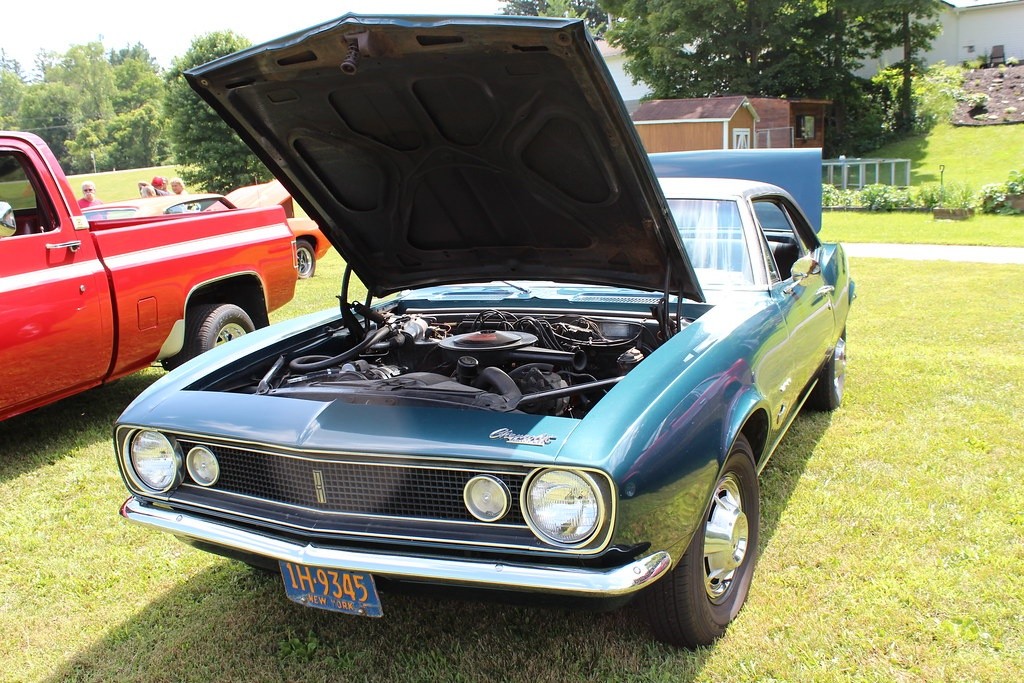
[0,131,298,423]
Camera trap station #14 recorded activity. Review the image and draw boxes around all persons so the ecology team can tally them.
[138,176,189,213]
[77,181,108,219]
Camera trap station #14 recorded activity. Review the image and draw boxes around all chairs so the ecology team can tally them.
[9,209,47,239]
[989,45,1006,66]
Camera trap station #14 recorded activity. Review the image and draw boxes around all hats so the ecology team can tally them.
[152,177,163,186]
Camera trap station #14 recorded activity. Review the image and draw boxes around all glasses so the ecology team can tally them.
[84,189,93,192]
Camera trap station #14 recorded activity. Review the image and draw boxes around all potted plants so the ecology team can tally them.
[932,188,972,221]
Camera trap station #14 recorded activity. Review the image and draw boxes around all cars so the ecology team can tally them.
[114,10,860,641]
[81,178,331,280]
